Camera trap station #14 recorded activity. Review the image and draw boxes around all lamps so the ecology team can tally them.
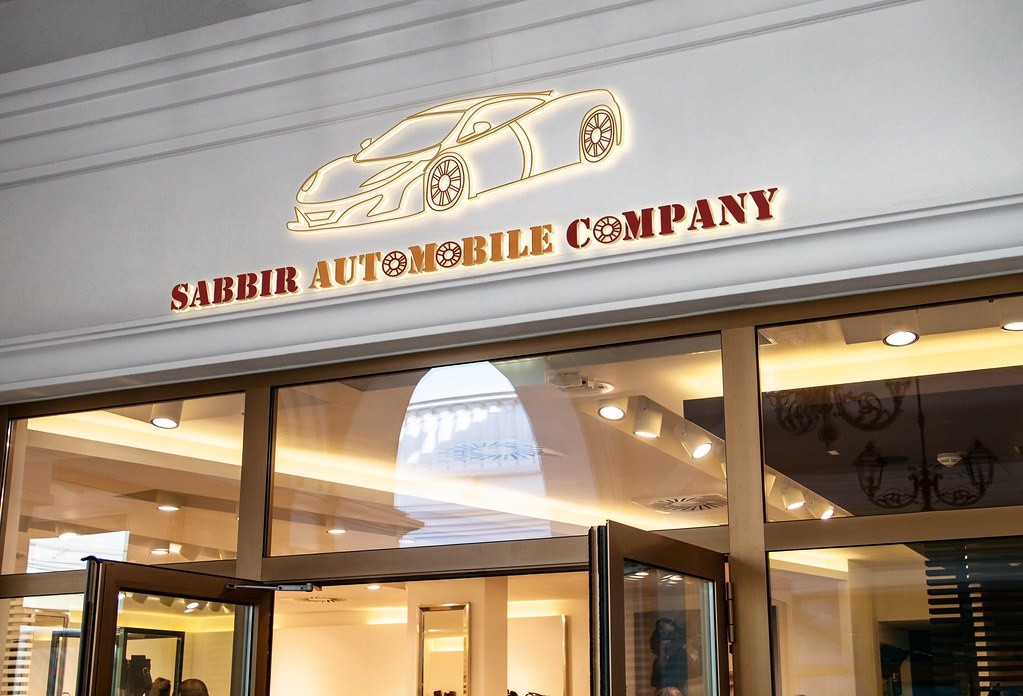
[881,309,920,347]
[151,401,182,429]
[632,406,664,438]
[854,377,997,512]
[997,297,1023,331]
[781,488,806,510]
[673,421,713,460]
[807,498,834,519]
[595,398,628,421]
[767,378,910,451]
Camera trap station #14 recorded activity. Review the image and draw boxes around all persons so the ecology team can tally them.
[147,677,209,696]
